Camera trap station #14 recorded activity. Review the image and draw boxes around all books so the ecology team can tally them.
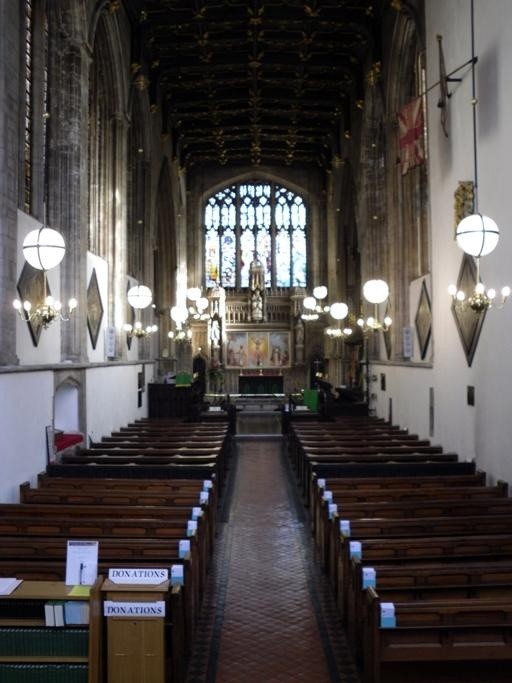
[0,576,24,599]
[43,601,91,629]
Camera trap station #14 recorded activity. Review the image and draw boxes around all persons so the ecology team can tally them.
[66,540,99,587]
[228,348,235,365]
[252,338,263,363]
[274,346,281,364]
[236,346,246,366]
[279,350,289,364]
